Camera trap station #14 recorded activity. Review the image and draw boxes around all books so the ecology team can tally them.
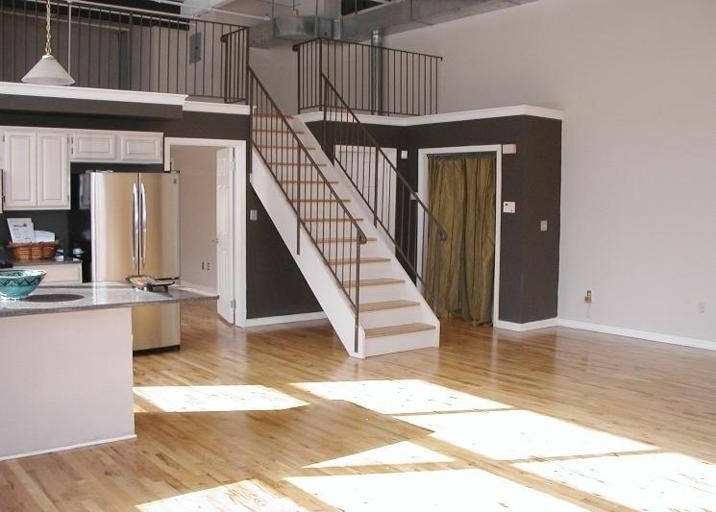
[6,216,31,221]
[32,230,55,242]
[8,222,33,243]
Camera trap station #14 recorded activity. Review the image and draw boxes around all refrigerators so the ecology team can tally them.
[89,169,184,357]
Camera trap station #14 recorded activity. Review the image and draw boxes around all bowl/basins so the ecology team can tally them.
[0,267,48,301]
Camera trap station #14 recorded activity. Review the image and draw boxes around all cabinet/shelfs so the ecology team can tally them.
[0,126,71,211]
[68,129,164,165]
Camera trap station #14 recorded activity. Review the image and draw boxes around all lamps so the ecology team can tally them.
[20,0,75,85]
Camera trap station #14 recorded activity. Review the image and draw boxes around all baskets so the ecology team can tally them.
[5,240,60,260]
